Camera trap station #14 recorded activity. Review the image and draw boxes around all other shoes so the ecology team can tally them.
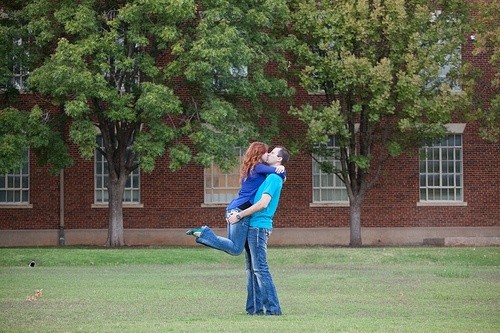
[185,228,204,237]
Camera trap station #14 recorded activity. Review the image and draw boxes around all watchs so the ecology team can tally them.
[236,213,242,220]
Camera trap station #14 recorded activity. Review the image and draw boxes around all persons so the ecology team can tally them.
[186,142,286,256]
[226,146,290,315]
